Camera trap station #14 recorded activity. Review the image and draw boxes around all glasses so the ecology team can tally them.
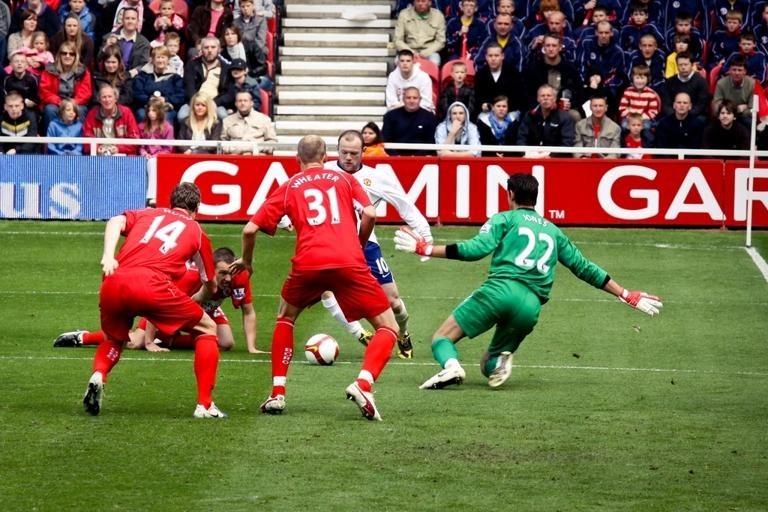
[60,51,76,56]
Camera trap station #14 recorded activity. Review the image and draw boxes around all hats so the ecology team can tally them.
[227,58,247,70]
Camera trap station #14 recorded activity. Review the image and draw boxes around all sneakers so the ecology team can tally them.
[259,394,287,414]
[83,370,104,416]
[359,330,413,361]
[193,400,229,420]
[53,330,91,348]
[344,382,384,422]
[487,350,513,388]
[418,366,466,391]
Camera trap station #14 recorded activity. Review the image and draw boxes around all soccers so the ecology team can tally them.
[305,334,339,365]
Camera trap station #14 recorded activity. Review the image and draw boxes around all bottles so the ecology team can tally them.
[507,110,521,123]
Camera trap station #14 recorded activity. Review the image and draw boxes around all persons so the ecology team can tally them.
[381,0,768,158]
[228,134,401,423]
[2,1,277,158]
[277,129,434,361]
[360,122,390,157]
[392,173,664,390]
[52,246,272,355]
[82,182,231,420]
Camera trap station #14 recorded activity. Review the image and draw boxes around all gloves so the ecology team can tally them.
[619,288,663,316]
[393,225,434,257]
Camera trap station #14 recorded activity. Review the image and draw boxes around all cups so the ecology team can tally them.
[559,98,570,110]
